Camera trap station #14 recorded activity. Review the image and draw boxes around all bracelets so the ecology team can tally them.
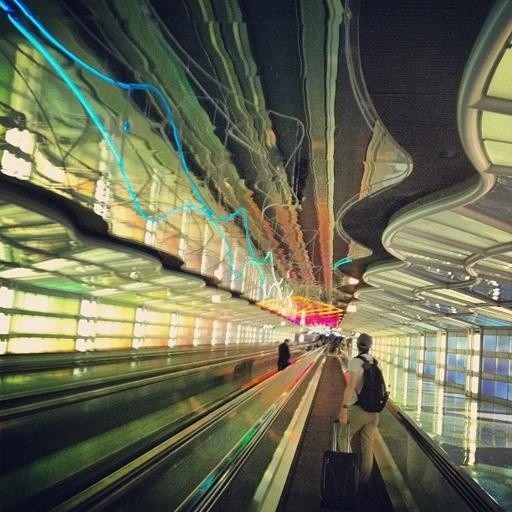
[343,404,348,408]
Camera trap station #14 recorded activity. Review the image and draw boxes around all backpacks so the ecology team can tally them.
[355,355,390,412]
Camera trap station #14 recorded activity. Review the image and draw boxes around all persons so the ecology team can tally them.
[336,333,384,494]
[278,338,290,371]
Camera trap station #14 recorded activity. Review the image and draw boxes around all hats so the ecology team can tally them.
[357,333,373,349]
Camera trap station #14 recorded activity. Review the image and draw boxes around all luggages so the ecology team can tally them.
[318,419,361,509]
